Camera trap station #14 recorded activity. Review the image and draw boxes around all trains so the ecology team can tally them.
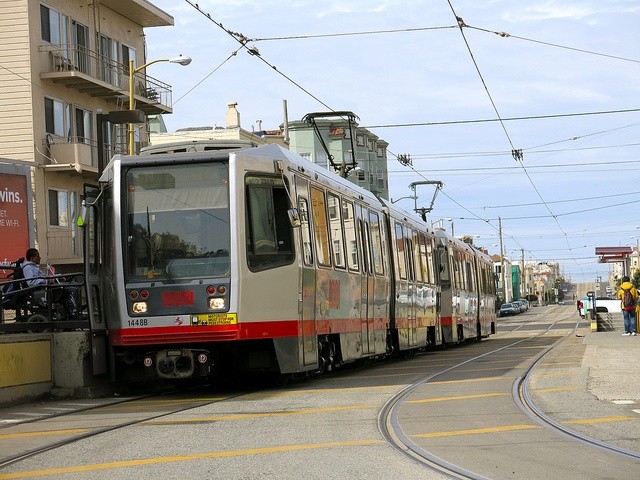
[77,141,499,390]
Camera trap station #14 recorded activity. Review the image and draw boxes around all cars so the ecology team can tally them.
[513,303,520,314]
[559,300,564,305]
[500,304,514,317]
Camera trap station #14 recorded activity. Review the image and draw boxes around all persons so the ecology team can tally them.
[616,275,639,337]
[21,248,89,318]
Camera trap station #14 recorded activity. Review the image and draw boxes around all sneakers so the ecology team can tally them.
[632,331,636,336]
[622,332,631,335]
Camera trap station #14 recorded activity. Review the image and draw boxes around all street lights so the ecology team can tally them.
[129,54,192,155]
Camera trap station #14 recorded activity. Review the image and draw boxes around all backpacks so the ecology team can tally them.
[621,286,635,307]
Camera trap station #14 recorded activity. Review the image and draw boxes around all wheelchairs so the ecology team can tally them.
[7,258,87,333]
